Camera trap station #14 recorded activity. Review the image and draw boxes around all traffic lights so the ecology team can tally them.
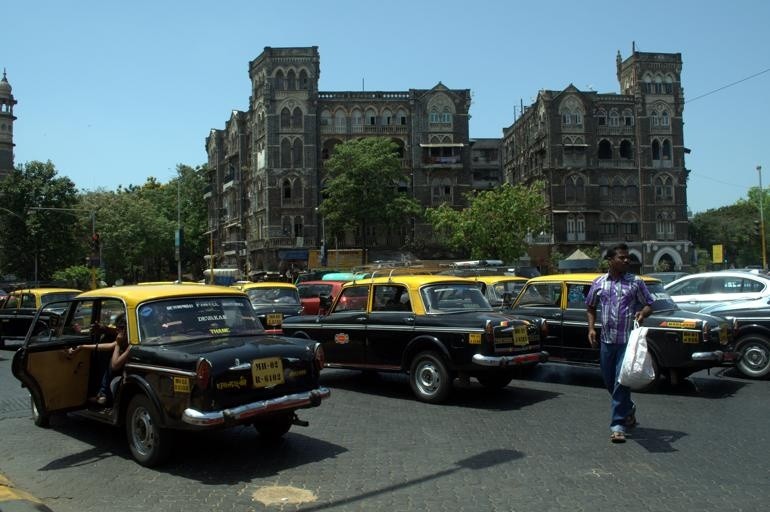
[753,218,762,241]
[91,232,100,253]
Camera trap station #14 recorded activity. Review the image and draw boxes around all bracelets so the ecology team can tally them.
[95,342,98,352]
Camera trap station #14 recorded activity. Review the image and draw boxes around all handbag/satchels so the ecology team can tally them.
[618,318,656,389]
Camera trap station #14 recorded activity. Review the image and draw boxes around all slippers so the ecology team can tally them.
[625,413,636,425]
[610,430,628,444]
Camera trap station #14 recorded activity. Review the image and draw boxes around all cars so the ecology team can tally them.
[9,279,335,468]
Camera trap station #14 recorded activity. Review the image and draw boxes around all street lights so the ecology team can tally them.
[313,205,328,268]
[755,163,768,269]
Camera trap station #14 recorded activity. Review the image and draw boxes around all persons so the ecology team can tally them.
[584,243,655,443]
[97,277,107,287]
[68,312,130,405]
[285,266,304,283]
